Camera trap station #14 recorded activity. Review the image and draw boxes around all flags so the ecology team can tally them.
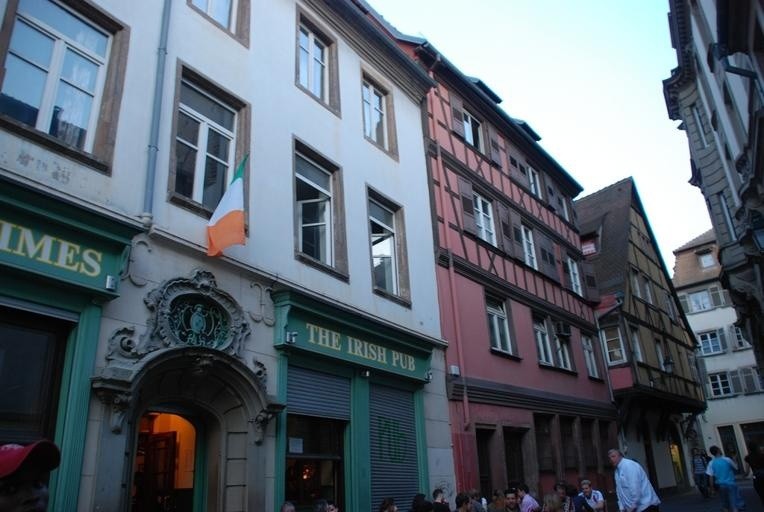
[204,155,245,257]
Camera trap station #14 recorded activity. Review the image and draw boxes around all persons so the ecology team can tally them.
[281,480,604,512]
[691,440,764,512]
[608,449,660,512]
[0,437,61,512]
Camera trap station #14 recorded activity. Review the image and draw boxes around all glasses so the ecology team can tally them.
[504,488,518,496]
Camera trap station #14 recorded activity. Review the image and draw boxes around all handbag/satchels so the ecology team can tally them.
[694,485,701,495]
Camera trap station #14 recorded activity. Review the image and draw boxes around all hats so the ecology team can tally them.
[0,439,63,480]
